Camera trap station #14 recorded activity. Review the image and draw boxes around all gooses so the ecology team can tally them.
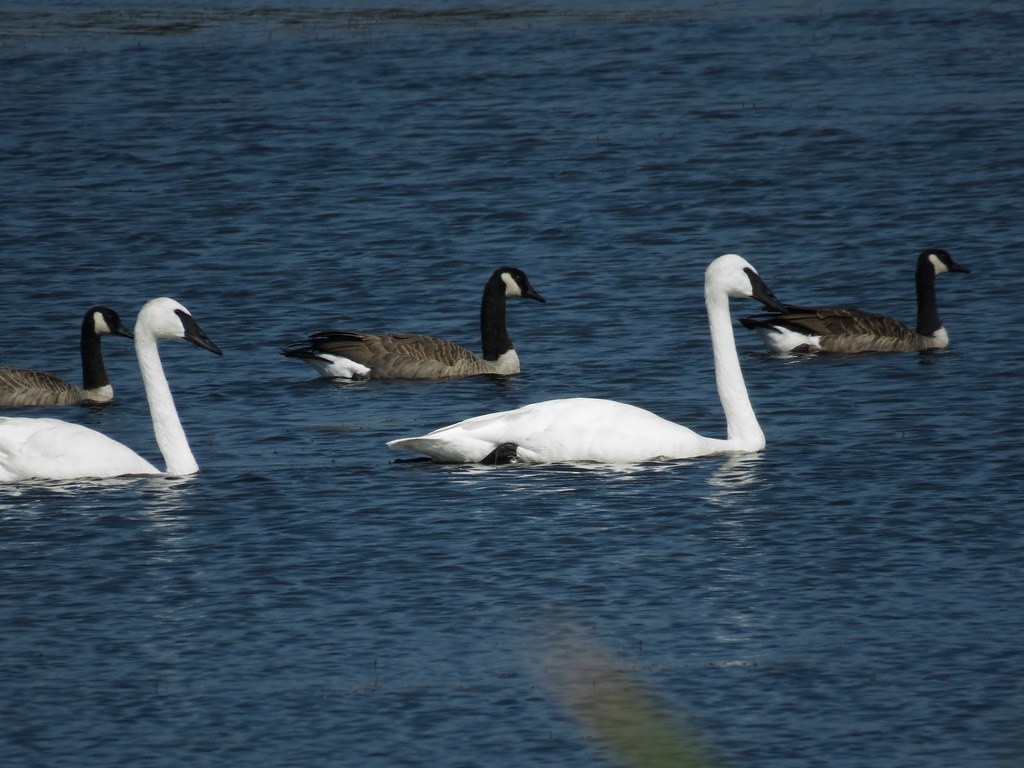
[384,254,790,466]
[739,248,973,356]
[1,298,225,485]
[0,307,135,408]
[278,269,549,385]
